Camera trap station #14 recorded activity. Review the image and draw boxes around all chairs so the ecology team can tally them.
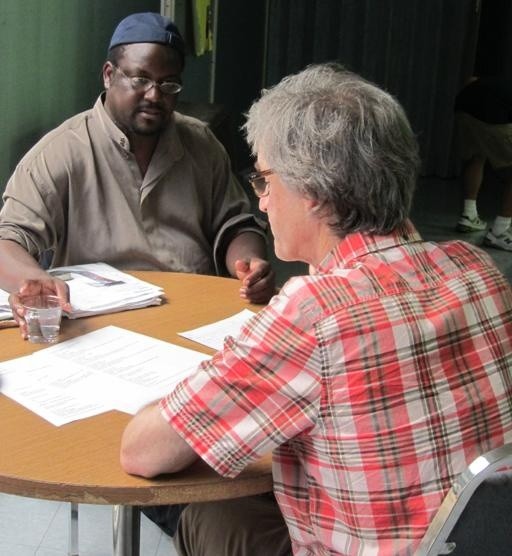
[411,444,512,556]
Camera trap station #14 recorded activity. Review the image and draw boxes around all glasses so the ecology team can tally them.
[130,77,182,95]
[247,168,274,198]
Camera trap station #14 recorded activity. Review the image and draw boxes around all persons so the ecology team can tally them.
[1,11,278,535]
[452,0,511,251]
[120,62,511,556]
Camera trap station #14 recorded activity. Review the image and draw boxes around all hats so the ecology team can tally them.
[108,11,186,51]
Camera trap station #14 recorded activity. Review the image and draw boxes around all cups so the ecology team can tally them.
[19,293,66,344]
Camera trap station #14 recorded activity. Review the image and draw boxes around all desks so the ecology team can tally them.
[0,270,274,556]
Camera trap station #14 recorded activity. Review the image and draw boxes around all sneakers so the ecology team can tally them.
[485,228,512,251]
[456,215,487,231]
